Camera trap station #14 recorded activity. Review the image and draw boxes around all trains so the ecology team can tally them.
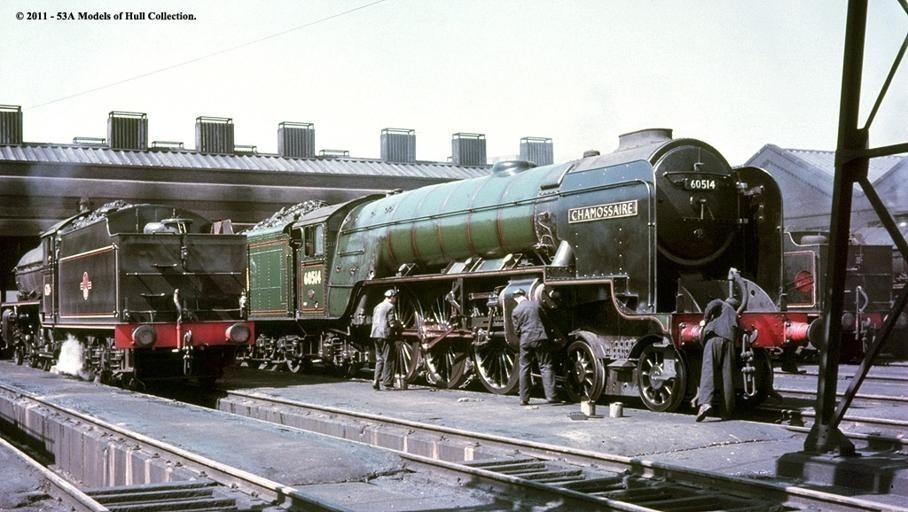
[239,126,907,412]
[1,198,257,390]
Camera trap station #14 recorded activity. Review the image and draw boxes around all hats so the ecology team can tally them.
[514,289,526,294]
[726,297,737,305]
[384,290,398,296]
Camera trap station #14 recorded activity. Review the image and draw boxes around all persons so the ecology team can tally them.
[695,295,740,421]
[510,288,562,405]
[368,289,403,390]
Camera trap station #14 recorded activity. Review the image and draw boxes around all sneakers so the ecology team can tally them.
[372,379,394,390]
[696,405,712,422]
[520,401,527,405]
[549,399,565,404]
